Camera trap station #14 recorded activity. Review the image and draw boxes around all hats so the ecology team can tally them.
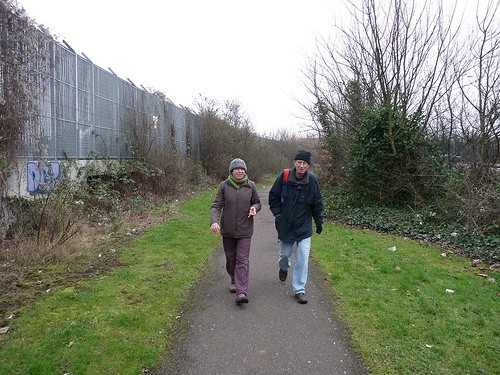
[294,150,311,164]
[229,158,247,173]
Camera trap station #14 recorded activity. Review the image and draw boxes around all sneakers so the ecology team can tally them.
[294,292,308,304]
[229,283,236,293]
[235,294,249,305]
[279,268,287,281]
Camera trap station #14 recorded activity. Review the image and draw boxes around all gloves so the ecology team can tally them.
[316,225,322,234]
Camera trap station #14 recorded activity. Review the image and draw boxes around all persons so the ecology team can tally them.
[268,149,325,304]
[208,158,262,305]
